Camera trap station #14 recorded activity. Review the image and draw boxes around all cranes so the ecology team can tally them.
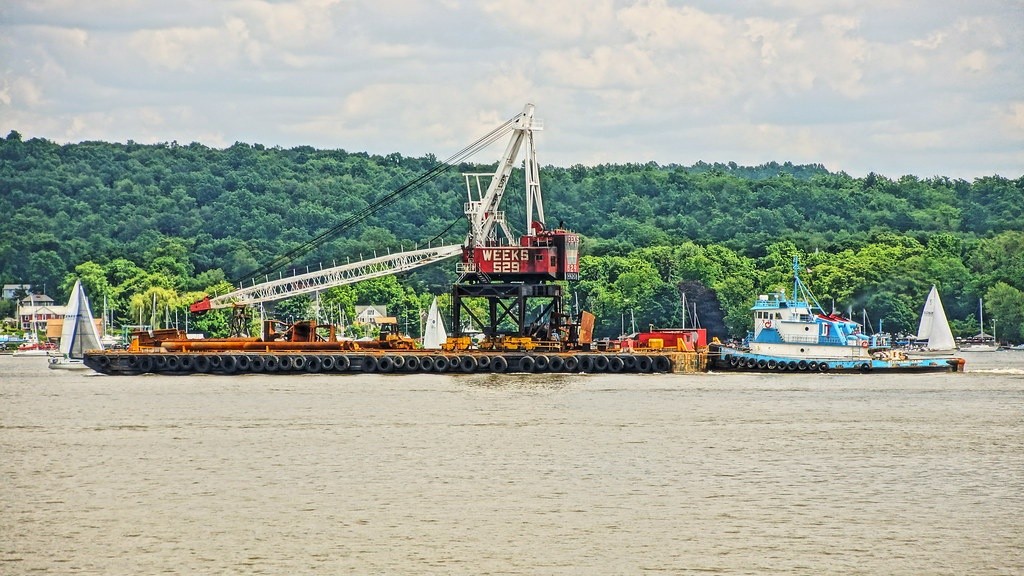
[190,102,579,342]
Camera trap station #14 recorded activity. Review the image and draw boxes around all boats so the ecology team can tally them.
[707,249,966,376]
[83,289,710,374]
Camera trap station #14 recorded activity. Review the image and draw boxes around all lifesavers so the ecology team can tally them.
[548,356,564,373]
[98,355,111,368]
[636,356,653,373]
[518,356,535,373]
[594,355,609,371]
[652,355,671,373]
[127,355,139,367]
[861,363,870,373]
[724,353,830,374]
[563,356,578,372]
[608,356,624,373]
[765,322,772,328]
[577,355,594,373]
[624,355,637,368]
[362,353,510,375]
[861,341,869,348]
[535,354,550,371]
[137,356,351,373]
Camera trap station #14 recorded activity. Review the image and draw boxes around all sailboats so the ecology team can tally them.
[862,284,1024,354]
[13,290,61,357]
[47,279,111,372]
